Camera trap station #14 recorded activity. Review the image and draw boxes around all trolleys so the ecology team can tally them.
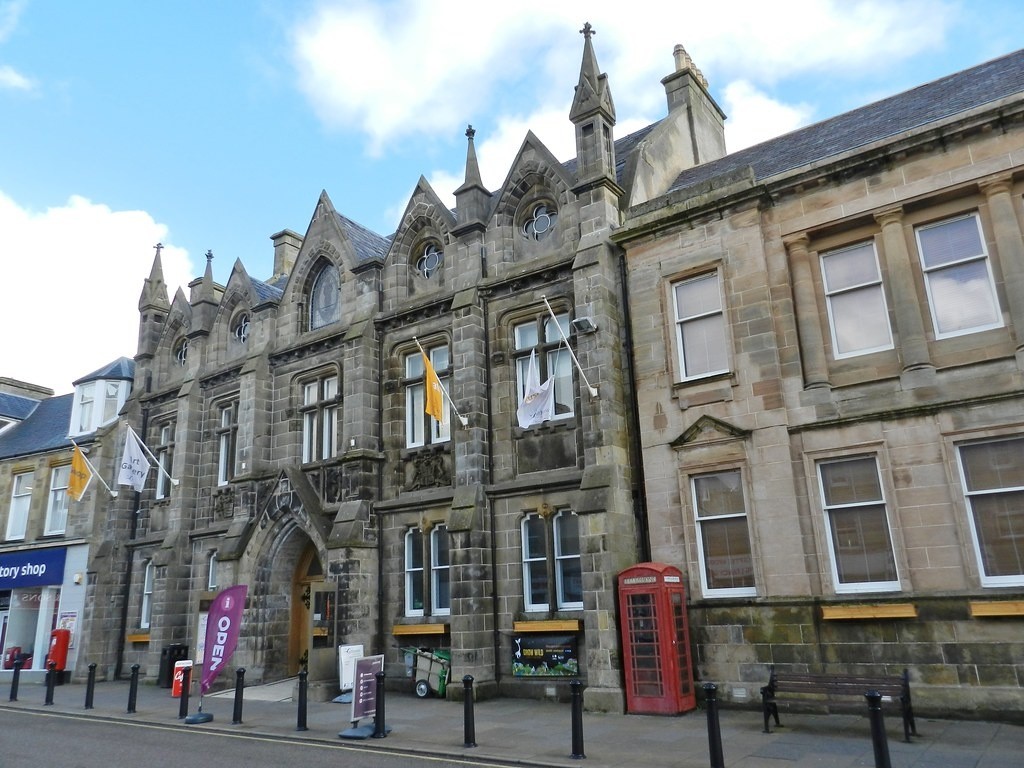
[401,645,450,699]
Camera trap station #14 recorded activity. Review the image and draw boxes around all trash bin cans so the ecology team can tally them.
[157,642,188,689]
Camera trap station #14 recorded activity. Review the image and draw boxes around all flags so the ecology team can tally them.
[424,353,442,422]
[66,446,93,502]
[117,428,151,493]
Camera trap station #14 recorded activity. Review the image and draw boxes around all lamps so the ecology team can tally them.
[72,448,90,455]
[571,316,598,336]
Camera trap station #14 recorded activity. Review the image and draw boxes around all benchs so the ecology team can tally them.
[760,666,917,744]
